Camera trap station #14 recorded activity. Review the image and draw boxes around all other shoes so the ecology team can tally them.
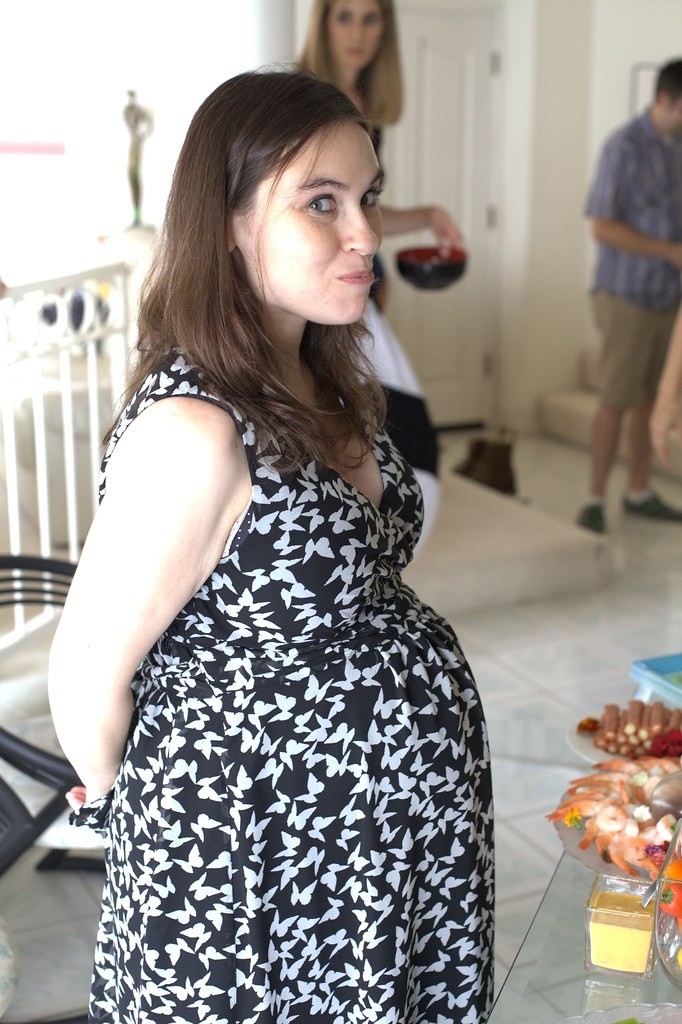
[579,505,602,531]
[623,494,680,522]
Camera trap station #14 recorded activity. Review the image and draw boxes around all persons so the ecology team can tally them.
[576,56,682,533]
[46,73,496,1023]
[294,0,467,545]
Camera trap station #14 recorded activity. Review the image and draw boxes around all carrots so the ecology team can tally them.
[664,857,682,881]
[660,883,682,923]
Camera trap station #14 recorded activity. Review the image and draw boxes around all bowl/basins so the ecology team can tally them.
[397,246,466,289]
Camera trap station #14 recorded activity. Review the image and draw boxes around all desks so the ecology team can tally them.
[483,850,682,1024]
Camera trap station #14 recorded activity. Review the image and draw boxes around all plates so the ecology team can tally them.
[554,770,654,882]
[566,711,632,765]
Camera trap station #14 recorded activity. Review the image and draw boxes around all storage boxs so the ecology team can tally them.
[583,874,657,982]
[629,652,682,709]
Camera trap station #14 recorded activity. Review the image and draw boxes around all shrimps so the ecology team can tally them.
[547,755,682,880]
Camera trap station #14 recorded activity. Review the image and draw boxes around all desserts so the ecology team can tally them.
[593,700,682,757]
